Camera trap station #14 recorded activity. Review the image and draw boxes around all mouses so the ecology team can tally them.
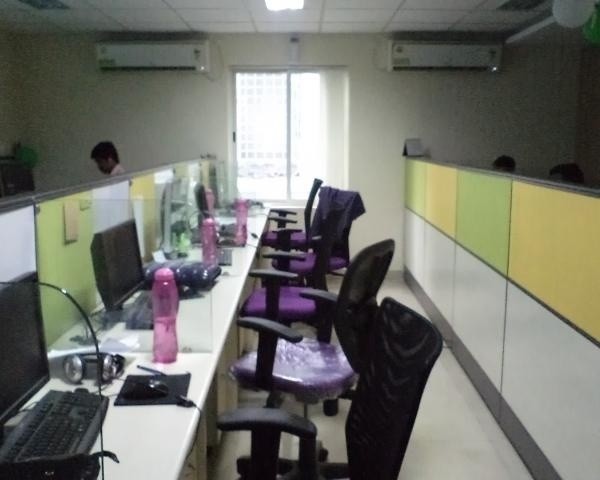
[179,284,194,297]
[178,252,188,258]
[123,379,168,399]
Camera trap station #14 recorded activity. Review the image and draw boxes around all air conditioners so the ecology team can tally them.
[94,40,210,74]
[388,38,503,75]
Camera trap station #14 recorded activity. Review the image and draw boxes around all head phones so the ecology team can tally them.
[63,351,126,383]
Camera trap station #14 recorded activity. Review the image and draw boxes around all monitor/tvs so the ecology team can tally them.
[89,218,146,322]
[160,181,175,260]
[193,183,209,218]
[0,270,51,447]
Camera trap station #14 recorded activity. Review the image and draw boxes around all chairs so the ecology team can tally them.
[239,208,344,417]
[215,297,443,480]
[232,239,394,463]
[273,187,359,287]
[262,201,344,292]
[260,179,323,253]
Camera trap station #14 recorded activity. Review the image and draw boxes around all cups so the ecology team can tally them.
[234,197,248,246]
[152,268,179,363]
[202,218,218,267]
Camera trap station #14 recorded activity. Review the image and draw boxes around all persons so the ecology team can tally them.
[548,160,586,187]
[90,139,124,177]
[492,154,519,173]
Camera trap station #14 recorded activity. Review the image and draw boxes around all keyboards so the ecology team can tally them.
[126,292,154,329]
[0,389,110,479]
[216,223,248,248]
[217,249,232,265]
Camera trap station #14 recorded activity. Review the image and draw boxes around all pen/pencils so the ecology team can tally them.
[136,364,167,378]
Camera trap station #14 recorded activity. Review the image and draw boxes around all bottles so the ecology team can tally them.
[204,188,214,214]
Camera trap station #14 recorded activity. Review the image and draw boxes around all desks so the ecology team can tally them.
[0,202,268,479]
